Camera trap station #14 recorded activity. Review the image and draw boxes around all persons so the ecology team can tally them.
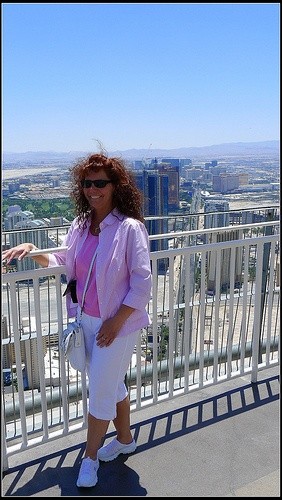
[1,139,154,486]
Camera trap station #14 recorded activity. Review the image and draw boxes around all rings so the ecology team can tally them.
[101,337,106,340]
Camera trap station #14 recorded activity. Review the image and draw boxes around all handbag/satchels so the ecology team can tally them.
[61,320,86,372]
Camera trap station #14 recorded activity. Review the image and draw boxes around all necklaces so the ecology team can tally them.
[94,227,100,233]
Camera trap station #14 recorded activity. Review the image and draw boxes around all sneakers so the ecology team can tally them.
[97,436,137,462]
[76,456,100,487]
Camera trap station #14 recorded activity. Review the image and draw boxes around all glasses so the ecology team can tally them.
[81,179,111,188]
